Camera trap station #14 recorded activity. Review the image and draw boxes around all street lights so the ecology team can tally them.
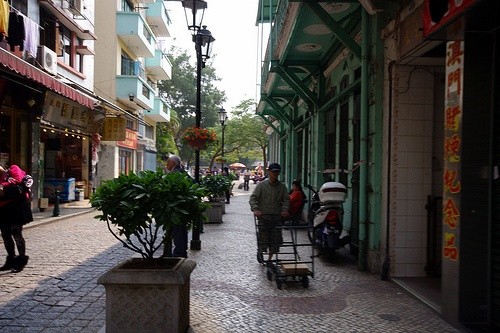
[181,0,215,251]
[216,107,228,174]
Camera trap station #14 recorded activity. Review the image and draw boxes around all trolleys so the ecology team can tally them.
[254,213,315,290]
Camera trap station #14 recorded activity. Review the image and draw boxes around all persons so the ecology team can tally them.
[0,165,33,272]
[252,163,261,175]
[287,181,306,219]
[223,167,232,204]
[250,163,290,263]
[167,155,188,258]
[244,168,251,190]
[190,167,240,179]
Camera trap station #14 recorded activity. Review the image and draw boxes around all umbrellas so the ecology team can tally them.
[229,163,246,168]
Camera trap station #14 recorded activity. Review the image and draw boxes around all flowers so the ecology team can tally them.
[180,127,220,150]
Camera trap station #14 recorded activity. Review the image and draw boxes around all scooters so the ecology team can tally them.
[302,182,349,263]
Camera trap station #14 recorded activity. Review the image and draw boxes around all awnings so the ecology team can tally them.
[74,83,151,128]
[1,47,95,110]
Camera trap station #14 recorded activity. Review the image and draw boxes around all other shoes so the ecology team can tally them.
[12,256,29,273]
[267,262,276,269]
[0,256,15,271]
[257,251,264,263]
[225,202,230,204]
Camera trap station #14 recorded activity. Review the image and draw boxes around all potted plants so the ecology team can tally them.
[87,167,214,333]
[198,174,236,223]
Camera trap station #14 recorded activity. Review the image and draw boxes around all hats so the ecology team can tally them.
[269,163,281,172]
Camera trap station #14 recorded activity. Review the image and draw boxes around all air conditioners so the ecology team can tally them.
[34,45,58,76]
